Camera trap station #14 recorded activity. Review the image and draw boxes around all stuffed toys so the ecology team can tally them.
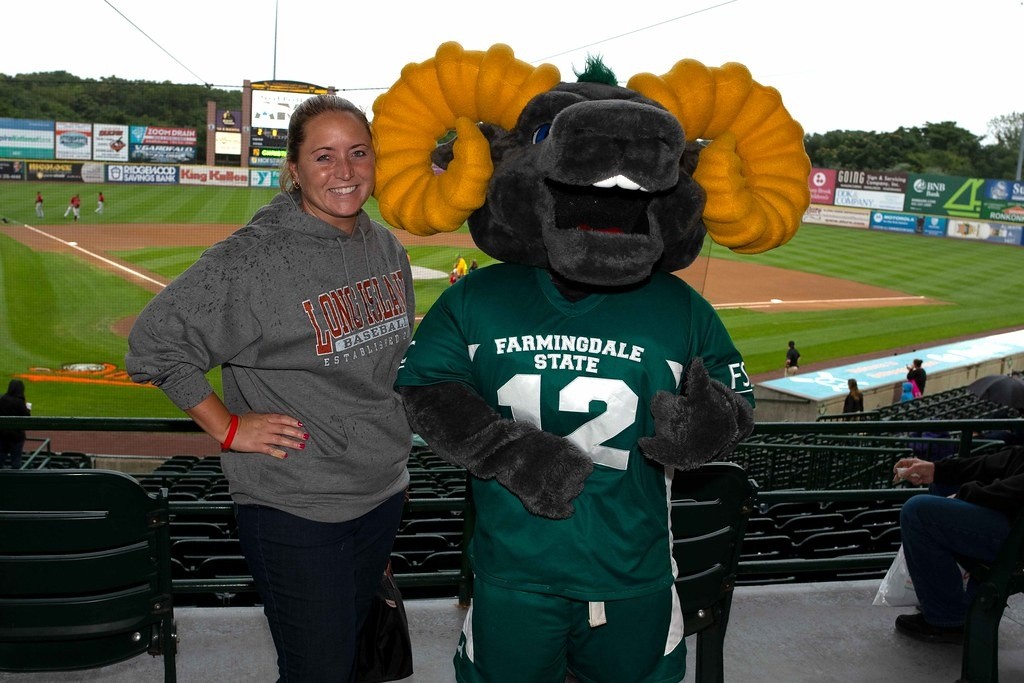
[372,41,812,682]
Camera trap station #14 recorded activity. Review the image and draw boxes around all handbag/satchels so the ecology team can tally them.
[357,570,413,683]
[872,542,969,607]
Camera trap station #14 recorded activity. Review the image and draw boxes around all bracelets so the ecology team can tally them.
[220,414,239,450]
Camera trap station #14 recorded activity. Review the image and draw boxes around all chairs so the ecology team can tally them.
[958,574,1024,682]
[670,461,762,682]
[0,450,266,683]
[386,371,1023,600]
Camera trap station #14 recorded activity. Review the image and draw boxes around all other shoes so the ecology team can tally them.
[895,612,964,645]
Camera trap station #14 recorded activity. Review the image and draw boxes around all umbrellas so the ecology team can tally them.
[967,374,1024,415]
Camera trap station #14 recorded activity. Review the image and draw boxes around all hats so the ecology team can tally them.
[914,359,923,367]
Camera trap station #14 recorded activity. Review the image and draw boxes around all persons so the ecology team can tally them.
[892,443,1024,644]
[95,192,105,216]
[906,359,926,398]
[785,341,801,377]
[899,382,915,402]
[126,97,419,682]
[910,416,1024,461]
[64,193,80,221]
[842,379,864,421]
[0,380,32,470]
[35,192,45,219]
